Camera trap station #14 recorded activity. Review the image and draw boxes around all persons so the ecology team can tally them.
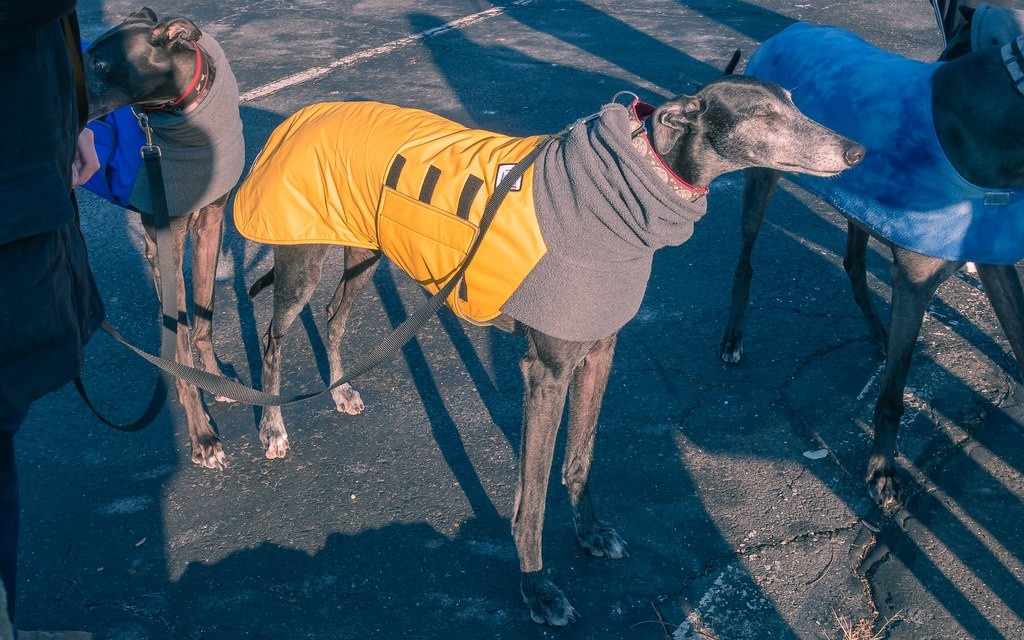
[0,0,104,640]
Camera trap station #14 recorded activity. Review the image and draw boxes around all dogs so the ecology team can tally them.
[80,6,1023,627]
